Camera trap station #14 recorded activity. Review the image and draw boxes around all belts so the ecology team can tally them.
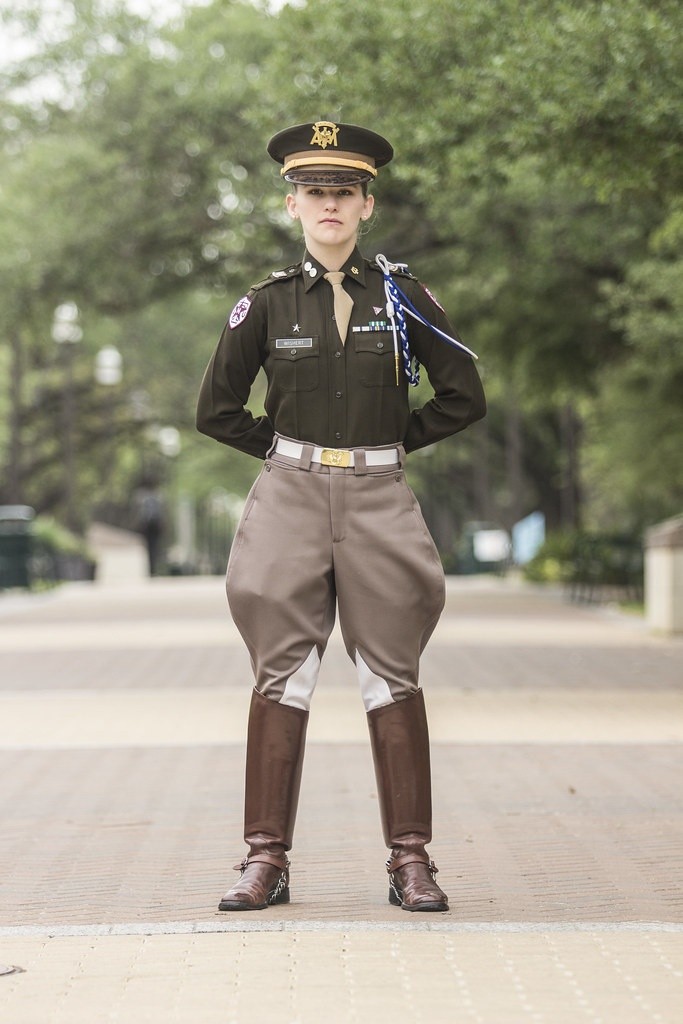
[273,438,398,468]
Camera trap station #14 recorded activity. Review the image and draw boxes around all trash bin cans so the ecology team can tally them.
[641,513,683,632]
[0,504,36,588]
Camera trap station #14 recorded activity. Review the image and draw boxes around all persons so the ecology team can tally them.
[196,119,486,911]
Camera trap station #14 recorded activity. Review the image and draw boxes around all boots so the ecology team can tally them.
[218,686,311,911]
[365,686,450,912]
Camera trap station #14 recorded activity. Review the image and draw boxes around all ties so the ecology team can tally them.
[322,272,354,347]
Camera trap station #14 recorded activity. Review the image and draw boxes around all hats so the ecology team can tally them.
[266,121,394,187]
